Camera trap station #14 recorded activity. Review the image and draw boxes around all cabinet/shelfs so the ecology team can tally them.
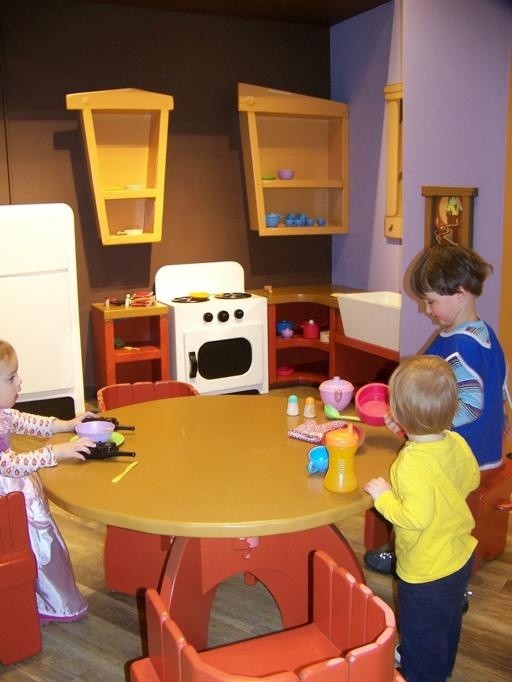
[65,86,175,248]
[91,299,169,397]
[245,284,400,401]
[237,81,349,237]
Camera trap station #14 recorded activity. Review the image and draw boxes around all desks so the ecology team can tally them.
[38,394,407,653]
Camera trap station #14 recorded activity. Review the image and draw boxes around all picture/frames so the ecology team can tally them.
[421,185,478,252]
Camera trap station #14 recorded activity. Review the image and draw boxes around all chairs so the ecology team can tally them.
[363,454,512,577]
[0,491,42,666]
[128,549,406,681]
[96,380,200,413]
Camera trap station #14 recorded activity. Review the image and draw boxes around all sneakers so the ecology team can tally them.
[364,544,395,576]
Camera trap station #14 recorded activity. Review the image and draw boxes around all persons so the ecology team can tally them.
[381,243,509,610]
[364,356,483,681]
[0,337,100,623]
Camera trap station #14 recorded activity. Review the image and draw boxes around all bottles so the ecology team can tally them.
[323,423,359,494]
